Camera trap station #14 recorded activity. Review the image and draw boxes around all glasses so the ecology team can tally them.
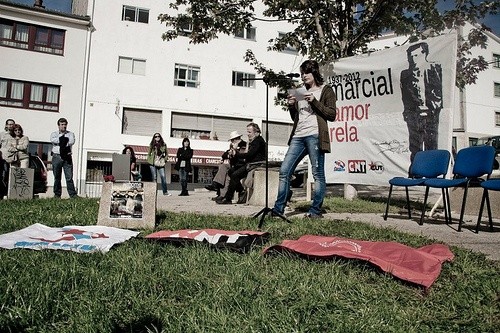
[154,135,160,138]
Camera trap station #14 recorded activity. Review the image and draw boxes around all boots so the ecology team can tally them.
[178,190,189,196]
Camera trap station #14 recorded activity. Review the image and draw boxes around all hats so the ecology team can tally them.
[228,131,242,142]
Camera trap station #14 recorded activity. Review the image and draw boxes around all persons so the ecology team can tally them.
[174,138,193,196]
[204,122,267,204]
[8,124,30,169]
[146,133,172,197]
[271,60,336,217]
[0,119,15,200]
[50,118,79,198]
[121,146,137,182]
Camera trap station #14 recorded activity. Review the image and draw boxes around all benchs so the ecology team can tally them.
[241,166,280,206]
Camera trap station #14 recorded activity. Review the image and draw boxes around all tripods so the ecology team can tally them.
[239,77,296,229]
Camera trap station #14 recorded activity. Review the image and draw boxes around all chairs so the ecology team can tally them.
[384,147,500,233]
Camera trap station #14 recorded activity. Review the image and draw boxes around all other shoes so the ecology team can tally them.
[308,213,323,218]
[54,195,60,199]
[236,190,246,204]
[72,194,79,198]
[164,192,171,196]
[270,212,279,217]
[204,184,220,191]
[217,198,232,204]
[212,195,223,201]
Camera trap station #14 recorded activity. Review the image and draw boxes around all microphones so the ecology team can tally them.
[286,72,300,78]
